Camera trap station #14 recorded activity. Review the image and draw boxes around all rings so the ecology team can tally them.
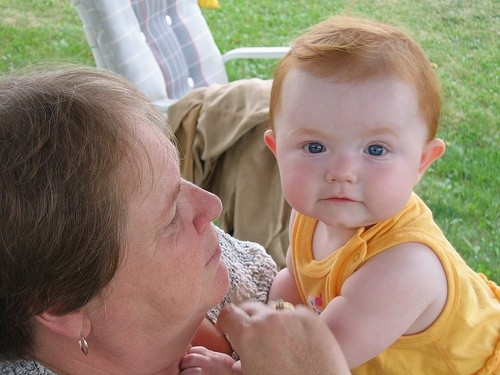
[275,298,295,311]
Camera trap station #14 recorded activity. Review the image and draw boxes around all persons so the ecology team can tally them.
[180,17,500,375]
[1,69,353,375]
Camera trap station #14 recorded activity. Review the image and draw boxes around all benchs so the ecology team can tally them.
[66,1,291,123]
